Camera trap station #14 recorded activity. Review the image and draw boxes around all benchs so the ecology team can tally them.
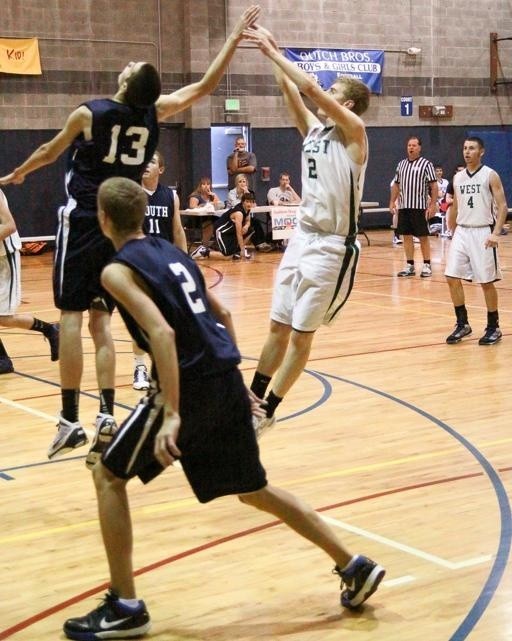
[363,208,396,229]
[19,237,55,243]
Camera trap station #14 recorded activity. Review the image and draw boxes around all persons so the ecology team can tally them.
[240,20,371,440]
[189,136,299,261]
[63,174,389,640]
[131,151,189,391]
[444,136,508,345]
[389,137,466,277]
[0,191,59,374]
[0,4,261,471]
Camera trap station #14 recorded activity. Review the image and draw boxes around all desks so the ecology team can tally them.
[179,201,379,252]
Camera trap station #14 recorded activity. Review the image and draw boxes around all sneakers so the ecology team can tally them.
[132,362,151,390]
[0,354,14,374]
[332,554,386,609]
[397,263,416,277]
[256,242,275,253]
[252,406,276,437]
[62,586,151,638]
[244,384,265,405]
[393,234,403,244]
[232,247,251,259]
[478,326,503,346]
[47,410,90,460]
[445,321,473,345]
[85,412,118,469]
[189,244,207,259]
[43,322,59,361]
[420,262,432,276]
[412,235,421,242]
